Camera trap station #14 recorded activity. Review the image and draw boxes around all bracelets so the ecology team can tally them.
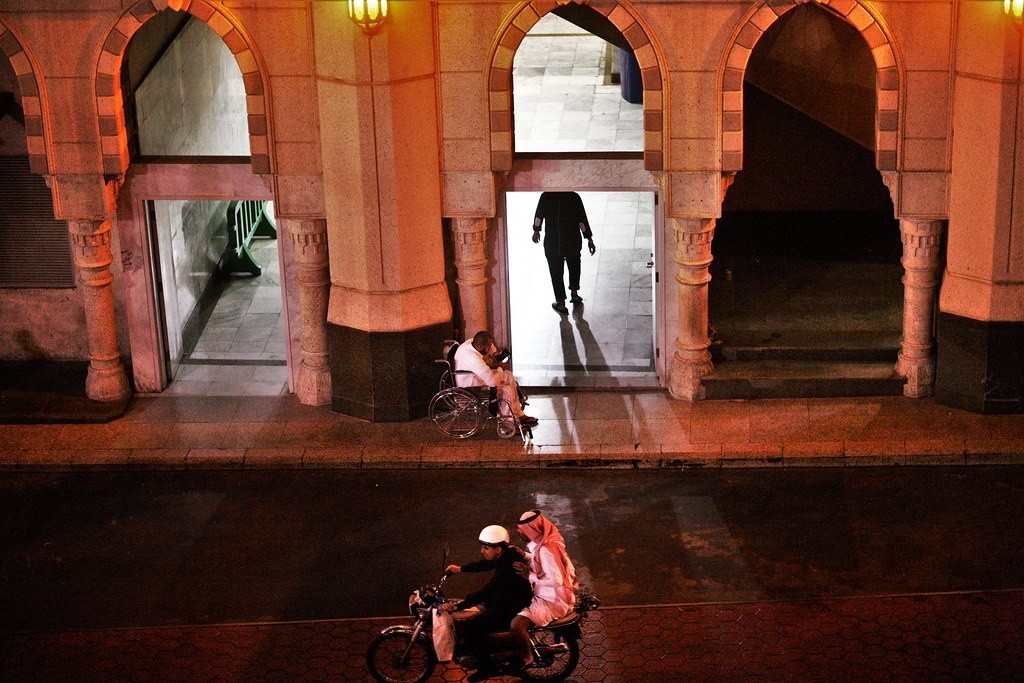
[588,238,593,241]
[524,551,525,556]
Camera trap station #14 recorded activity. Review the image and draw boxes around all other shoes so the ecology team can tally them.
[519,415,538,424]
[506,425,527,435]
[552,302,569,313]
[516,656,534,670]
[467,665,498,683]
[570,296,584,303]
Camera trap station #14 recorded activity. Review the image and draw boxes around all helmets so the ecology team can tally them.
[479,525,510,545]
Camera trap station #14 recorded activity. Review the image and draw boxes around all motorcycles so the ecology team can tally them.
[366,542,601,683]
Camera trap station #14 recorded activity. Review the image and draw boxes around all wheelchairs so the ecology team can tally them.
[428,339,534,443]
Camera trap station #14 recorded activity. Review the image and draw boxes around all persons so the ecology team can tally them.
[454,331,538,435]
[437,525,533,683]
[532,192,596,313]
[507,509,579,673]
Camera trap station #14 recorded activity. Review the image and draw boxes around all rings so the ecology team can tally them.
[520,568,523,571]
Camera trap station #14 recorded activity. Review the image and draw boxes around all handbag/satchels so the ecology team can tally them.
[432,607,457,663]
